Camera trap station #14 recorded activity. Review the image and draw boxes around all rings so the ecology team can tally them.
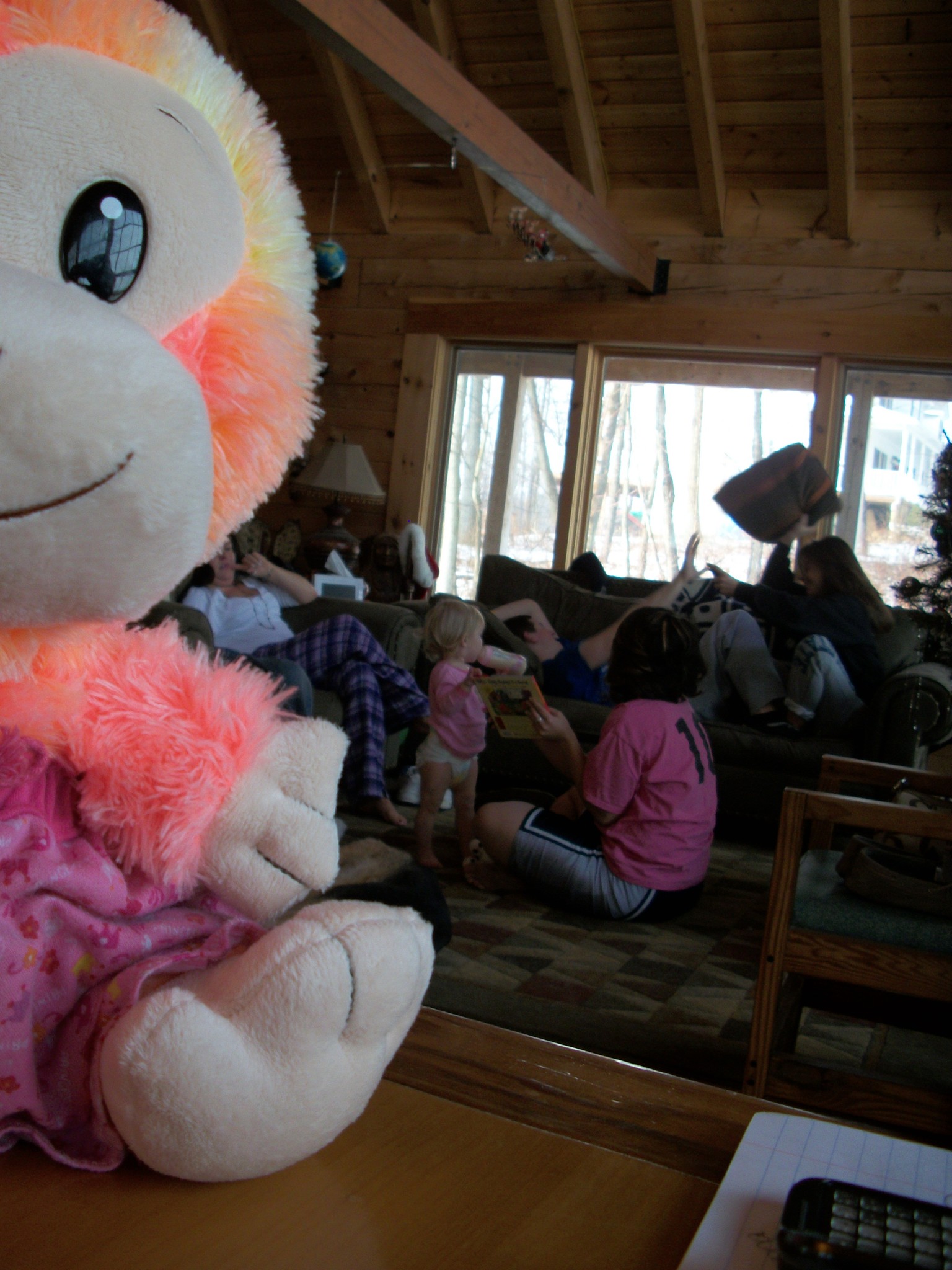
[535,717,542,724]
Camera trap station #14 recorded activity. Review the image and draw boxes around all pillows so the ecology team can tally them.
[714,442,846,546]
[671,579,776,650]
[564,552,616,595]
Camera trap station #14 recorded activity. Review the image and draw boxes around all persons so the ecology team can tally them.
[180,512,921,920]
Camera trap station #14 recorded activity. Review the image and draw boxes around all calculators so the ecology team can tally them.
[778,1173,952,1270]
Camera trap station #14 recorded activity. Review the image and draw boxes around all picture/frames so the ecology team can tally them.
[315,574,364,602]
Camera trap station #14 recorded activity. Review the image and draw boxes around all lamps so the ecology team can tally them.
[312,169,350,284]
[292,434,386,573]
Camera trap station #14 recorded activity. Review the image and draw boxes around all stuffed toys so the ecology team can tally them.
[1,0,437,1187]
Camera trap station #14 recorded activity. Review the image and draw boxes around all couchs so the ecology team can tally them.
[146,593,423,771]
[394,573,952,856]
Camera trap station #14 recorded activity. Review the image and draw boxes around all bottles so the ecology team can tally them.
[477,645,527,675]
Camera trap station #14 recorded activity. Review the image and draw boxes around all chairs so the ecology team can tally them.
[744,753,952,1147]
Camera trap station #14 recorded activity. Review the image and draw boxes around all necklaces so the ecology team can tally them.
[234,584,274,630]
